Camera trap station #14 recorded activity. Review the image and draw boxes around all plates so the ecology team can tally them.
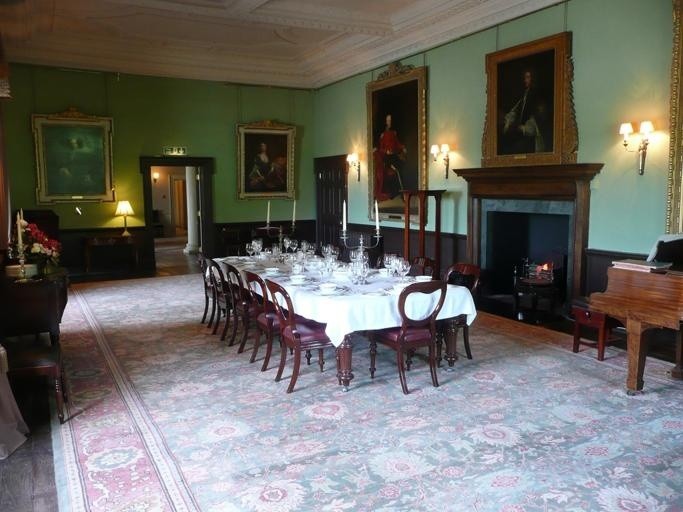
[5,264,38,279]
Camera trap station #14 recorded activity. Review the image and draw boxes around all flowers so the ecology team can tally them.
[21,217,62,279]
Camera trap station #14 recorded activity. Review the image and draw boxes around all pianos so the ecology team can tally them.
[589,260,683,392]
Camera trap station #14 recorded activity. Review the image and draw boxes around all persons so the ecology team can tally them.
[502,71,545,154]
[372,115,407,201]
[245,141,284,192]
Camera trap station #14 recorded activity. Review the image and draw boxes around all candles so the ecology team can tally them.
[20,207,25,220]
[293,199,297,225]
[266,200,271,223]
[343,200,347,231]
[17,212,23,244]
[375,200,380,230]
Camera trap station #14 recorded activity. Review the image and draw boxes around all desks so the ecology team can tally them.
[0,262,74,400]
[512,277,560,323]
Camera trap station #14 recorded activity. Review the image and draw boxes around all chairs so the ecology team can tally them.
[265,279,344,393]
[221,261,275,354]
[197,257,237,328]
[435,264,482,368]
[205,258,248,342]
[0,339,66,422]
[407,255,439,282]
[242,269,306,372]
[368,280,447,394]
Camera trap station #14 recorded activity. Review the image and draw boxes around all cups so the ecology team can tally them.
[252,239,262,257]
[350,251,370,286]
[319,244,341,284]
[284,238,290,255]
[383,253,411,284]
[245,243,254,263]
[290,239,298,254]
[301,240,317,260]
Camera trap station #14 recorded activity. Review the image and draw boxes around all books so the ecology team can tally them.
[612,259,672,273]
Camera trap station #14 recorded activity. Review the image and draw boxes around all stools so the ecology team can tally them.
[567,299,624,365]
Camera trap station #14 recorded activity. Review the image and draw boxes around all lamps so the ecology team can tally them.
[115,201,136,238]
[428,144,450,183]
[153,173,160,184]
[344,153,361,184]
[616,122,655,176]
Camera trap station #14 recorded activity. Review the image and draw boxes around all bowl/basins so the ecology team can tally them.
[289,274,307,283]
[265,267,279,275]
[244,262,257,269]
[378,268,394,277]
[319,283,337,291]
[415,275,432,282]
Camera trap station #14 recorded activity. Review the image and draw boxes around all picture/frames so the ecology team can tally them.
[483,32,578,167]
[234,119,297,200]
[364,60,427,227]
[32,110,116,204]
